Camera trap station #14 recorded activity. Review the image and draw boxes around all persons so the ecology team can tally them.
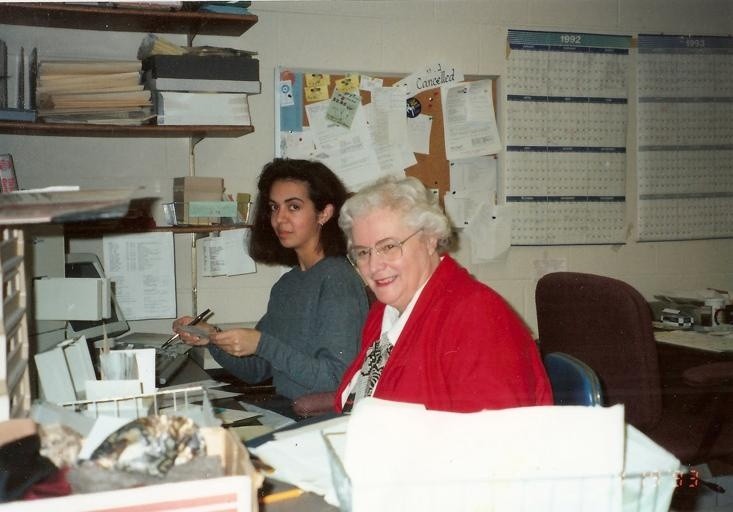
[171,158,375,402]
[331,172,556,415]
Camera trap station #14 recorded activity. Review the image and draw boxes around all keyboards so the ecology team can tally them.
[155,352,189,386]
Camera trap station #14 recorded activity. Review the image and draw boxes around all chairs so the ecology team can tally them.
[536,271,733,466]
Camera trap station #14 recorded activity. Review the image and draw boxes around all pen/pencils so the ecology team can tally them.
[259,487,305,504]
[161,308,212,348]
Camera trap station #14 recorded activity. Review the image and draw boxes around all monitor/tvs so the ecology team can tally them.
[64,252,131,342]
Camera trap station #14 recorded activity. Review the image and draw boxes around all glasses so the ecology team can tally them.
[346,229,427,263]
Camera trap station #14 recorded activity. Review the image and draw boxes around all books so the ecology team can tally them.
[32,55,157,128]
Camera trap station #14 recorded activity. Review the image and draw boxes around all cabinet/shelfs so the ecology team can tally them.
[2,6,261,243]
